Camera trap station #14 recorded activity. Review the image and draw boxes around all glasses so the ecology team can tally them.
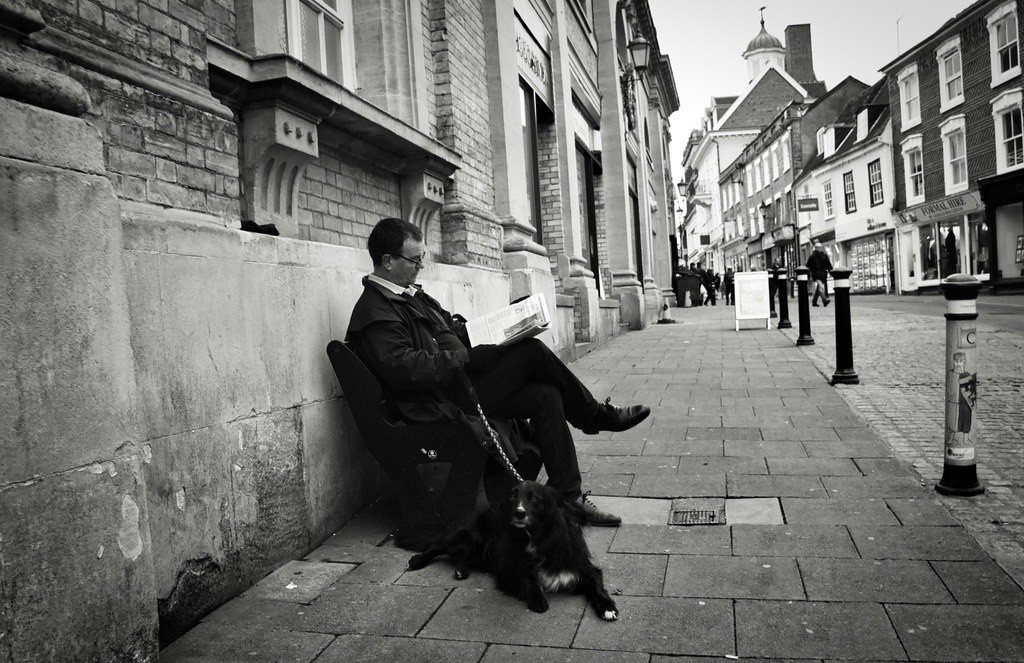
[401,251,426,269]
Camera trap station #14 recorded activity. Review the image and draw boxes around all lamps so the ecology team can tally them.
[664,178,688,204]
[759,200,773,220]
[620,27,651,86]
[665,206,686,220]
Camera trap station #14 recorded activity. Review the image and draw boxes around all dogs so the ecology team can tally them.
[407,481,619,622]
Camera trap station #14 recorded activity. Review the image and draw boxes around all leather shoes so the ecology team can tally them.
[574,490,622,526]
[582,396,650,435]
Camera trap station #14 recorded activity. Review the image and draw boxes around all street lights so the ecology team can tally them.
[675,206,684,258]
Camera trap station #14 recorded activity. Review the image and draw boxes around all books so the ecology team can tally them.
[465,290,555,352]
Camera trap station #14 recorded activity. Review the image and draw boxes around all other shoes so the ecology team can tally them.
[812,303,819,307]
[824,300,830,307]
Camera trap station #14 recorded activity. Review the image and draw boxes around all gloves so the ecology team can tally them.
[468,344,508,366]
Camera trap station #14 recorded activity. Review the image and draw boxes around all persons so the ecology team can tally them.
[771,257,781,301]
[703,267,718,306]
[737,266,742,272]
[714,271,720,291]
[690,262,701,306]
[806,241,834,307]
[723,265,735,305]
[749,264,757,271]
[695,261,709,305]
[344,218,651,527]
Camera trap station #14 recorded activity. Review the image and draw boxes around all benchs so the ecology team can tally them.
[328,337,542,547]
